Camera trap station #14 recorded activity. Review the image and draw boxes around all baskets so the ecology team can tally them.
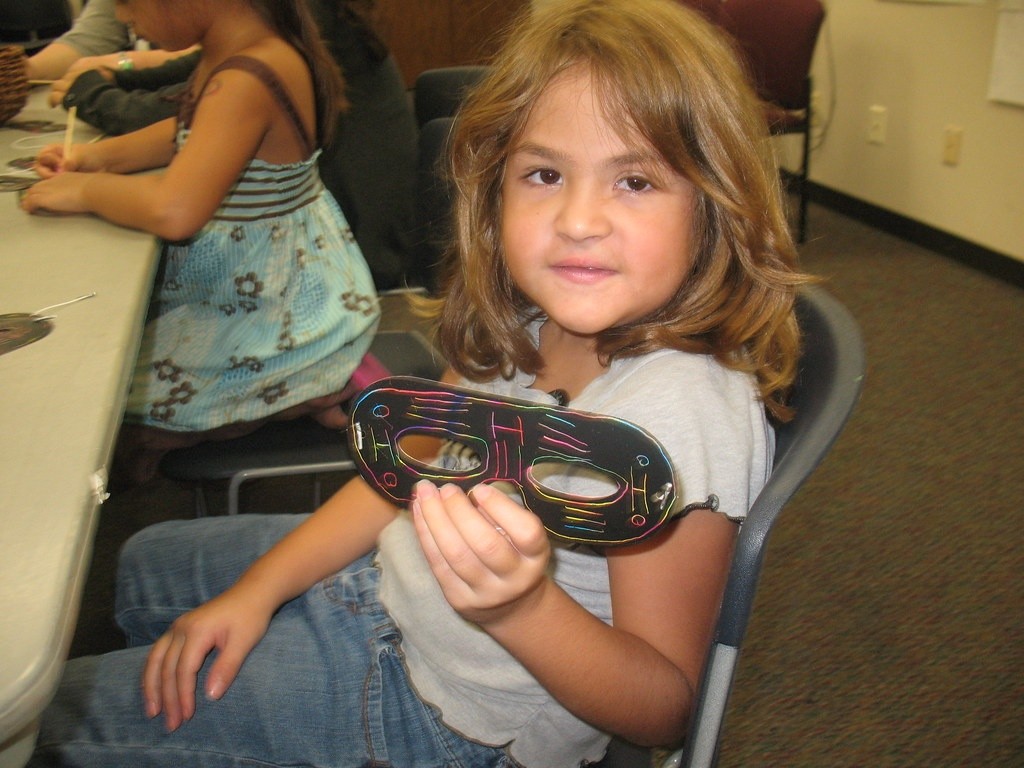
[0,44,28,124]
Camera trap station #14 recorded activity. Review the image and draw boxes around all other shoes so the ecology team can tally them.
[352,353,396,391]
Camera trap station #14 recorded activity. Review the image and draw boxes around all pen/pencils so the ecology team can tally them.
[57,106,77,172]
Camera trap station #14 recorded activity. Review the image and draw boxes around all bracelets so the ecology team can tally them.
[119,50,132,71]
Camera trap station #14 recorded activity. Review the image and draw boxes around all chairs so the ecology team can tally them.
[170,328,452,523]
[586,280,866,768]
[373,115,455,297]
[416,64,490,122]
[683,0,826,247]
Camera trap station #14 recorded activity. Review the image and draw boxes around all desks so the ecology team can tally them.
[0,82,166,768]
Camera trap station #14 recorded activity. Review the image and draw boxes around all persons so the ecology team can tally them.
[37,1,817,768]
[20,0,421,478]
